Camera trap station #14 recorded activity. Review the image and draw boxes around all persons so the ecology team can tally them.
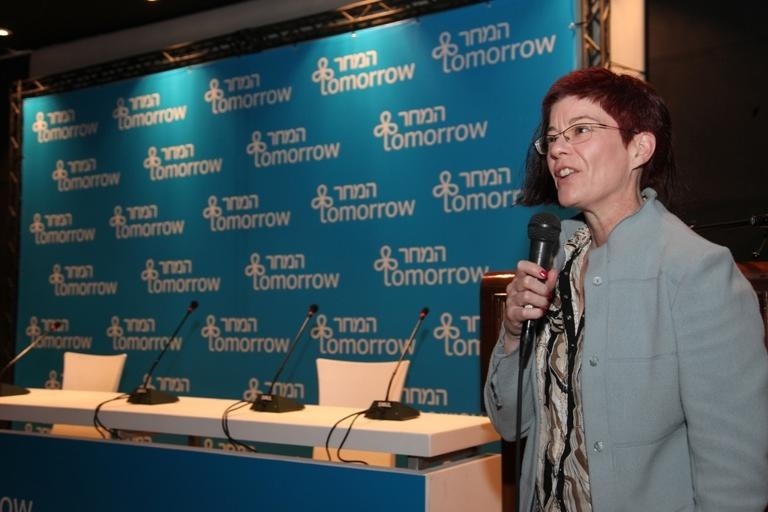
[484,65,765,510]
[508,118,693,227]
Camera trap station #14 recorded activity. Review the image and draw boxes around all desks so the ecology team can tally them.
[0,388,503,512]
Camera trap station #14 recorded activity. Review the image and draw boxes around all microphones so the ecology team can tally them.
[0,321,62,397]
[364,307,430,420]
[127,300,200,405]
[519,211,562,369]
[249,303,320,413]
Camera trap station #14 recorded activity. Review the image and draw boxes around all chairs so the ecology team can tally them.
[50,352,128,439]
[312,358,410,467]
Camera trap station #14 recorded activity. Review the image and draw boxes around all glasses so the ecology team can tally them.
[532,123,639,155]
